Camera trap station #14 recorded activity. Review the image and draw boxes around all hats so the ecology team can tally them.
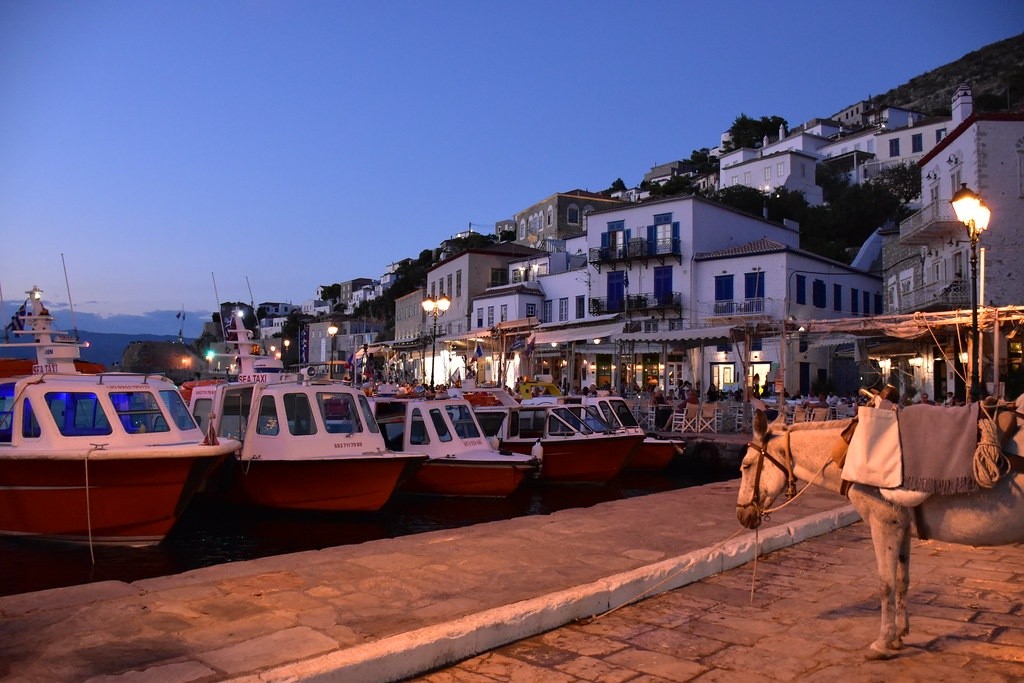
[654,387,662,392]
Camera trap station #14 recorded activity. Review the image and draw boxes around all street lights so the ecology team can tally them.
[283,339,290,372]
[951,182,992,403]
[327,322,339,379]
[421,292,451,391]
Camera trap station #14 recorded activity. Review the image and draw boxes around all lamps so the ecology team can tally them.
[637,361,643,371]
[301,367,316,381]
[879,356,891,368]
[927,247,938,258]
[908,351,922,369]
[926,170,936,180]
[947,154,958,165]
[947,236,958,246]
[590,362,596,374]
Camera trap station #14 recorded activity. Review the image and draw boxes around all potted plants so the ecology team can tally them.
[904,385,918,406]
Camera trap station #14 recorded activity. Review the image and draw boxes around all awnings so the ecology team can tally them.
[359,314,750,350]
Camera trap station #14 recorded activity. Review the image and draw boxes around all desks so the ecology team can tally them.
[658,404,674,428]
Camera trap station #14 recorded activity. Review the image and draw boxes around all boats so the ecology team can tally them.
[460,403,647,485]
[0,253,242,541]
[524,394,687,473]
[150,270,430,512]
[315,389,540,499]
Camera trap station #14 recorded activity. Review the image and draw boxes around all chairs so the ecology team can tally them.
[625,394,867,433]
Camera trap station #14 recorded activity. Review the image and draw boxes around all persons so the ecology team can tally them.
[344,369,957,431]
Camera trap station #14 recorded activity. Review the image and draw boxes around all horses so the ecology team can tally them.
[735,395,1024,662]
